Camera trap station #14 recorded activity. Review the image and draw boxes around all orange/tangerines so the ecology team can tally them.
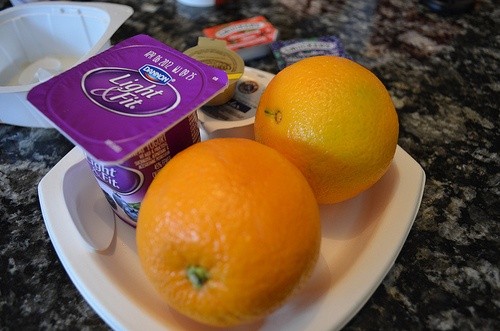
[255,55,398,204]
[136,138,322,326]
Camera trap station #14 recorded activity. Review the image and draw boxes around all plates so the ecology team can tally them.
[37,66,426,331]
